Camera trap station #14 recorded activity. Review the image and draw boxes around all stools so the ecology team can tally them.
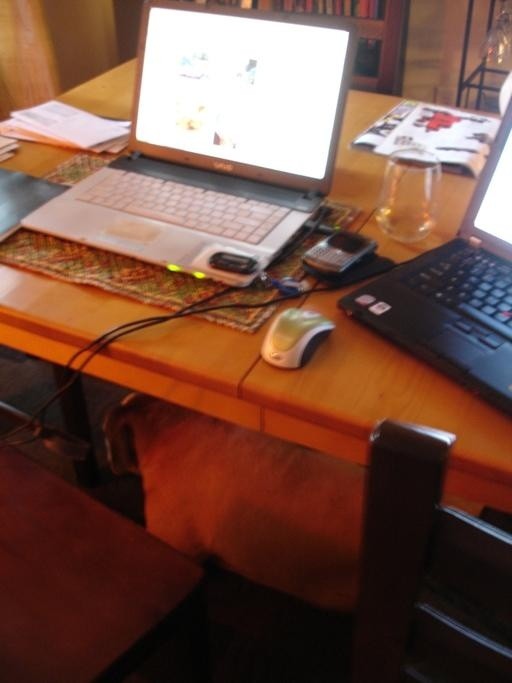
[3,442,206,682]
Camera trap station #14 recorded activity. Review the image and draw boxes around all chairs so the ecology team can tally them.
[345,418,509,682]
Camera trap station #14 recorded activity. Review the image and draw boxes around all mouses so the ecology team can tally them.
[260,307,336,370]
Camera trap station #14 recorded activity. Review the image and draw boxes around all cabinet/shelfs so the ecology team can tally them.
[255,0,407,94]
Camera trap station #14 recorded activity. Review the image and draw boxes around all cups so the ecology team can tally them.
[376,147,443,241]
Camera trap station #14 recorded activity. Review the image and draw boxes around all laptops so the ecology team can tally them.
[337,91,512,418]
[20,0,363,288]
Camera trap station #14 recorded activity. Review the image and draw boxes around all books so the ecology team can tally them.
[194,0,379,18]
[0,100,134,164]
[350,98,465,174]
[370,100,503,176]
[0,166,73,243]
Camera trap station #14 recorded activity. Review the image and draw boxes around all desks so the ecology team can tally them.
[3,57,508,622]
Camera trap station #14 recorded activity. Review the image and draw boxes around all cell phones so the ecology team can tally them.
[301,229,377,275]
